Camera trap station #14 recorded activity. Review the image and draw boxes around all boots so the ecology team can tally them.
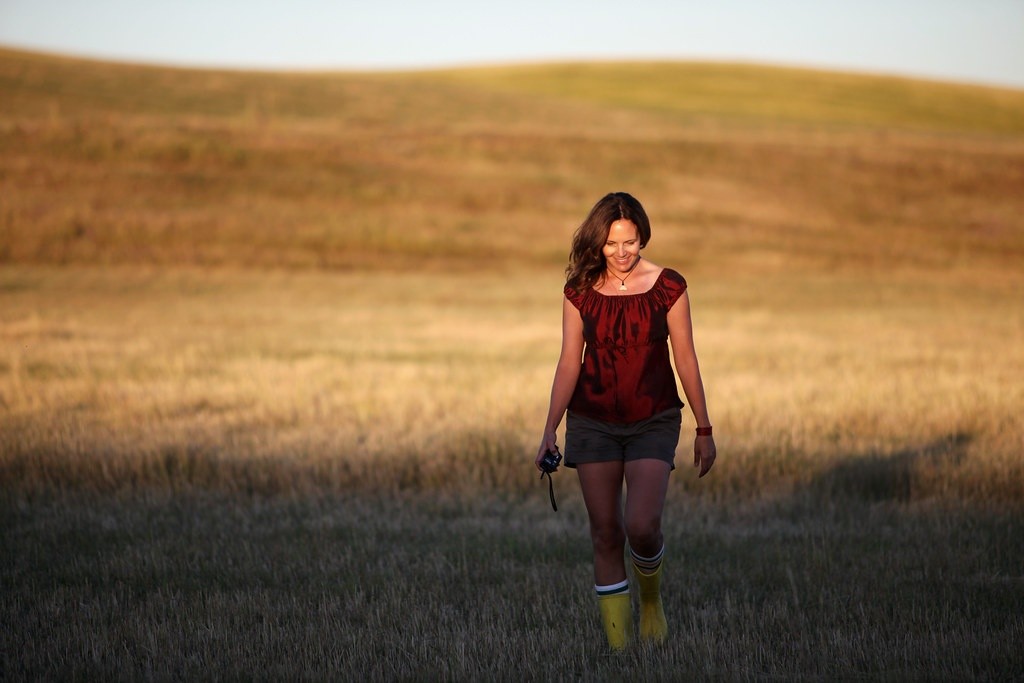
[596,594,632,652]
[631,558,667,647]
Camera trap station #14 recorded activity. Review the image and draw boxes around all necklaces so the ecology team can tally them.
[604,258,641,291]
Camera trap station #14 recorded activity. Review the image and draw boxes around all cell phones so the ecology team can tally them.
[539,448,562,473]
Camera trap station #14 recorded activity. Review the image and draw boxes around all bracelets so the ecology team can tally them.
[695,426,713,436]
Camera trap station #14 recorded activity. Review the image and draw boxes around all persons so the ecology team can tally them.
[534,190,717,652]
